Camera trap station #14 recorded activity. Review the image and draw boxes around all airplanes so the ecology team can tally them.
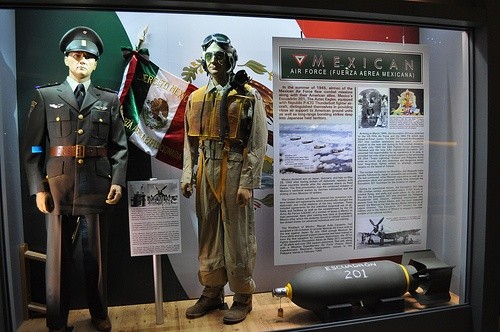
[360,217,421,246]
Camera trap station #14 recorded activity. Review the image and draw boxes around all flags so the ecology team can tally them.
[118,55,200,170]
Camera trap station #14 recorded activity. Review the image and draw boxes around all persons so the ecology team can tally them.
[133,192,172,207]
[22,26,128,332]
[181,35,268,325]
